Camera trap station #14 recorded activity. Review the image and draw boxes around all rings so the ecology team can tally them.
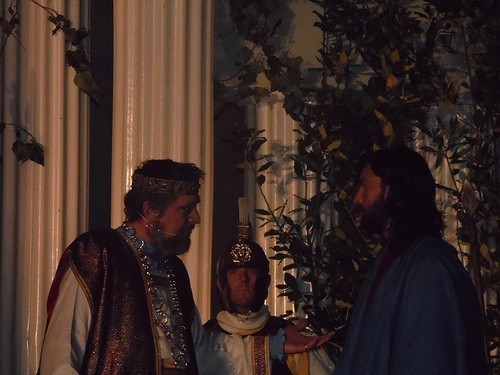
[304,344,308,350]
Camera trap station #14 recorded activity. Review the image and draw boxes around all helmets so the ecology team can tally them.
[216,197,271,313]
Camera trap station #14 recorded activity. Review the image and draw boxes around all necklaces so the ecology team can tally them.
[120,219,188,366]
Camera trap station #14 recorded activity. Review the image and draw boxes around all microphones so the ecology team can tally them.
[145,218,177,237]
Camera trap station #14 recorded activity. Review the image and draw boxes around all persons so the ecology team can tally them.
[36,158,334,375]
[332,144,494,375]
[200,214,340,375]
